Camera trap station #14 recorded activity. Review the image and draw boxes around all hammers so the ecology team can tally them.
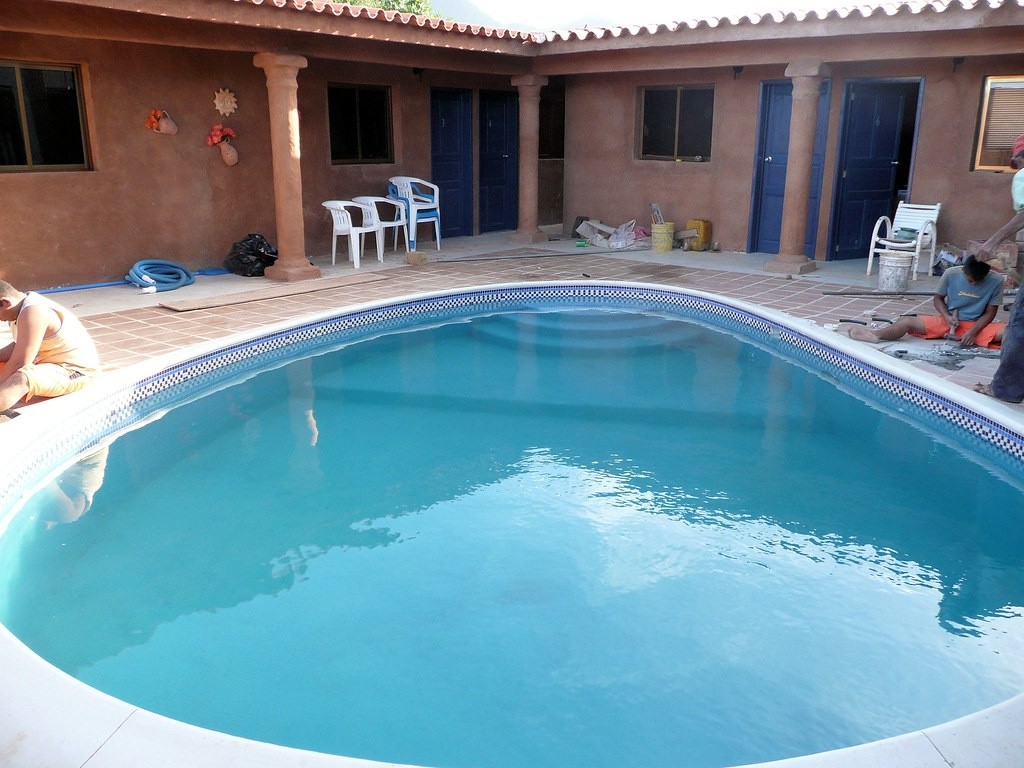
[943,308,960,341]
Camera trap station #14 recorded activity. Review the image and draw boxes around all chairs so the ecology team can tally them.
[351,195,409,265]
[867,200,942,280]
[321,198,384,269]
[388,174,441,252]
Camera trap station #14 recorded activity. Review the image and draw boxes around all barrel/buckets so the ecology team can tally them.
[687,219,712,251]
[878,254,912,292]
[932,242,964,274]
[652,222,673,252]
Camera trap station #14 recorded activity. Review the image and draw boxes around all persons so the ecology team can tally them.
[846,254,1010,350]
[975,135,1024,405]
[1,280,100,416]
